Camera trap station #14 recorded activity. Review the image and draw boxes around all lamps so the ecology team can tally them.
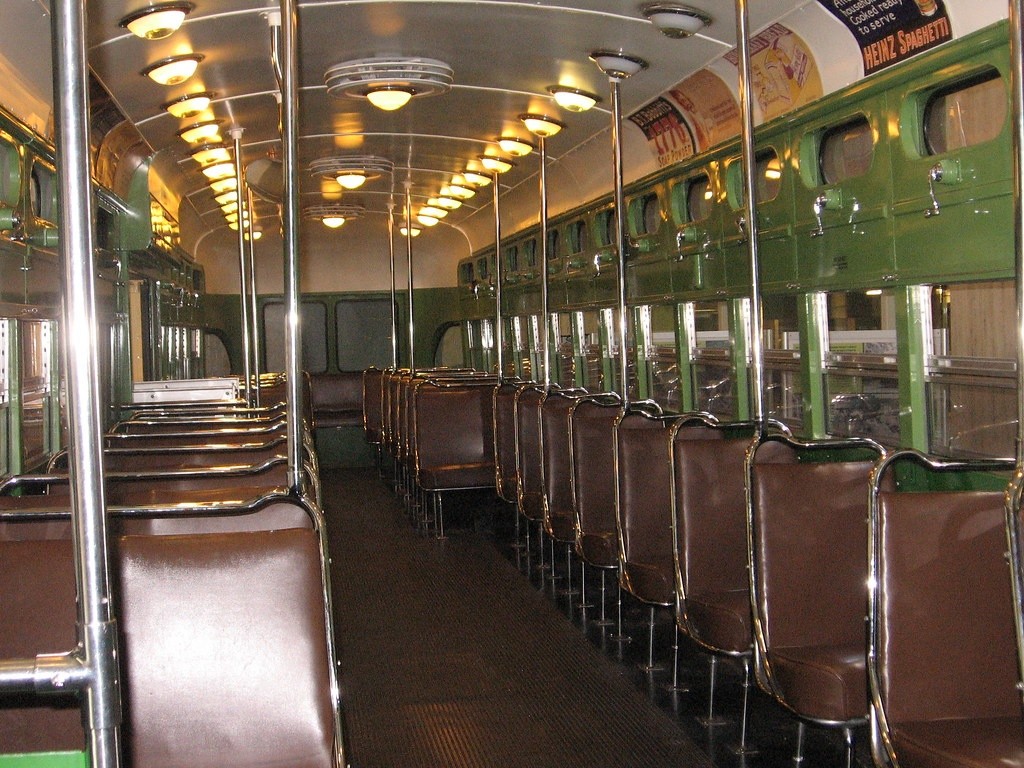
[399,2,714,235]
[321,215,345,228]
[334,173,370,188]
[366,86,417,112]
[119,1,261,241]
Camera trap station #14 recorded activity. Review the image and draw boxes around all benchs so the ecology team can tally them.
[1,367,521,768]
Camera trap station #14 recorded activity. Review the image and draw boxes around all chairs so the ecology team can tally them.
[492,379,1024,768]
